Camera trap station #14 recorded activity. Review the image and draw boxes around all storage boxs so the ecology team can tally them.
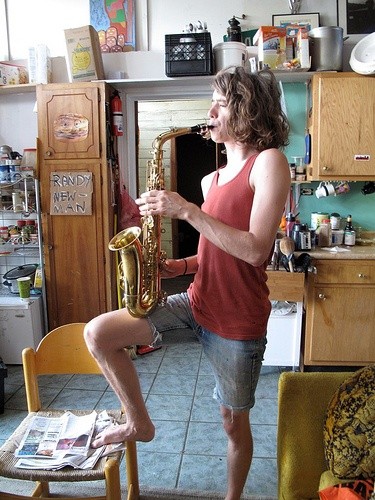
[63,25,106,81]
[252,25,287,70]
[164,32,216,77]
[287,37,293,62]
[297,29,312,72]
[28,42,53,84]
[0,61,28,86]
[107,71,125,79]
[20,148,37,170]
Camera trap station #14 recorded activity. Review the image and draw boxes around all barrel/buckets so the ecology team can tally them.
[0,159,34,182]
[310,212,329,231]
[308,26,349,71]
[213,41,248,74]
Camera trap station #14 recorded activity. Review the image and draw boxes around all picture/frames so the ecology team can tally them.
[336,0,375,46]
[272,12,321,44]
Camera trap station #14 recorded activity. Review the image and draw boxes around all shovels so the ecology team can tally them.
[280,237,295,271]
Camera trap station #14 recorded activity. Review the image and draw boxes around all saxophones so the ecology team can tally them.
[108,122,215,318]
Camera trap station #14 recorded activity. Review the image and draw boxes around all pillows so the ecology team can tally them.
[321,363,375,482]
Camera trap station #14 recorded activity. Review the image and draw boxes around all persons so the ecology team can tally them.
[84,65,292,500]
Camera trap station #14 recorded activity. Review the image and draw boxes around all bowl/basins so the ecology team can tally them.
[356,239,374,246]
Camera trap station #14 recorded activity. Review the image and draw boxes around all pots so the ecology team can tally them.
[2,264,39,293]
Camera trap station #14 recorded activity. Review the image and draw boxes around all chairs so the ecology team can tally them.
[276,372,359,500]
[0,321,141,500]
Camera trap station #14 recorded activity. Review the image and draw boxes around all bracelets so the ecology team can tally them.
[183,258,188,275]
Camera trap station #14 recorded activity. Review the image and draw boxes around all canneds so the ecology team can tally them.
[0,219,38,246]
[311,212,329,230]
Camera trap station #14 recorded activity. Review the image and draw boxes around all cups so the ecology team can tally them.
[345,231,356,246]
[16,277,31,298]
[315,182,349,198]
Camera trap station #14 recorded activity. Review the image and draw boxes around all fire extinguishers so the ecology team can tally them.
[110,86,124,135]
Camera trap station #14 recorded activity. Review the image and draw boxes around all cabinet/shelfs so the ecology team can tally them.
[305,71,375,183]
[260,265,305,367]
[291,243,375,373]
[0,171,48,338]
[34,81,123,332]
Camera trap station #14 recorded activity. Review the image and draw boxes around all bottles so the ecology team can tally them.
[286,214,295,238]
[0,219,36,241]
[344,214,353,231]
[292,224,312,251]
[228,16,242,42]
[27,193,35,212]
[318,224,332,246]
[12,193,22,213]
